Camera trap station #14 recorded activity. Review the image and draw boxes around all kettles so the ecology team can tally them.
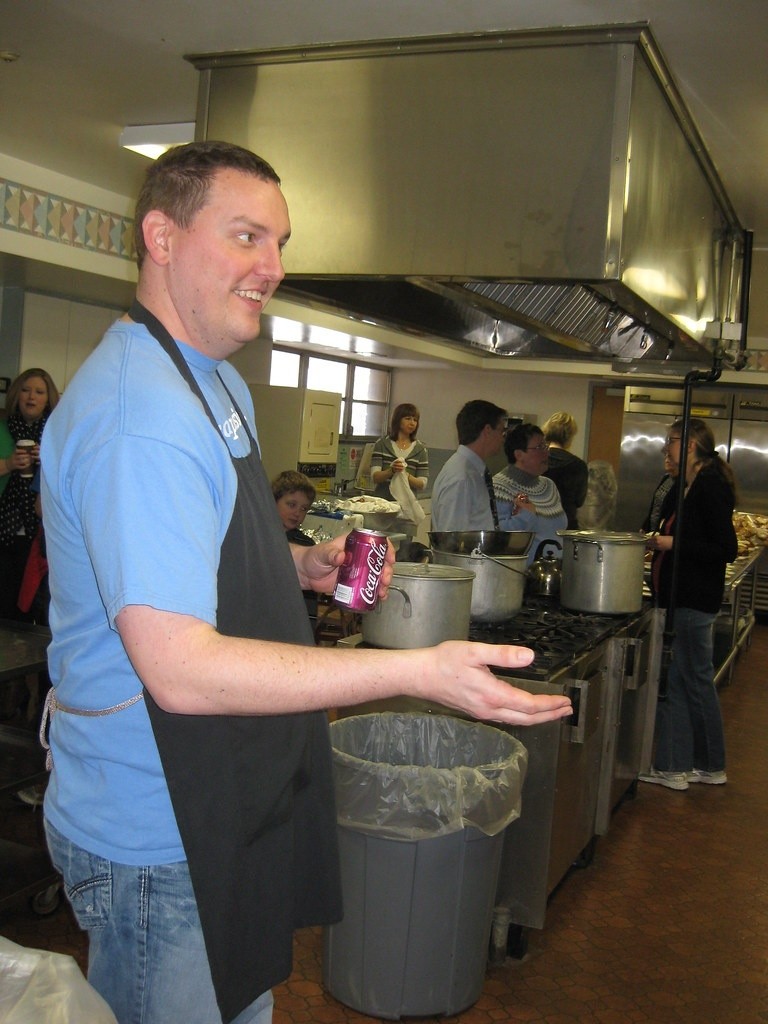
[523,538,565,595]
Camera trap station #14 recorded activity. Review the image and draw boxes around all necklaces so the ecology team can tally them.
[398,440,410,452]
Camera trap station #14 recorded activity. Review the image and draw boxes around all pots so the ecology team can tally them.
[357,561,477,650]
[430,546,528,622]
[557,530,650,616]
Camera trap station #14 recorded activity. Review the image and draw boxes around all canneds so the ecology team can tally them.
[510,493,529,516]
[331,527,389,614]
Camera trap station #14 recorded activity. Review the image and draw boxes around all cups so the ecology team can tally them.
[16,440,35,478]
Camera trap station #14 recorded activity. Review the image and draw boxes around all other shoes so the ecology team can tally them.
[18,782,45,807]
[34,881,62,914]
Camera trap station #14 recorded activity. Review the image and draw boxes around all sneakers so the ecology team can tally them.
[686,766,727,784]
[638,767,689,790]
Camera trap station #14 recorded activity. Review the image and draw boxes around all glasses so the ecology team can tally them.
[666,436,683,444]
[527,444,550,453]
[492,427,510,438]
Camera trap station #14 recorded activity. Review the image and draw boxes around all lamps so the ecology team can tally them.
[120,121,194,161]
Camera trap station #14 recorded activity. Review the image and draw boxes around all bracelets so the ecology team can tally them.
[408,473,410,479]
[392,467,395,473]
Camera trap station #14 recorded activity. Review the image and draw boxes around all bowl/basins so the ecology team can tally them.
[342,505,401,532]
[423,530,538,558]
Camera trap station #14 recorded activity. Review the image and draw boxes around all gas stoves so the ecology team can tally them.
[354,598,650,680]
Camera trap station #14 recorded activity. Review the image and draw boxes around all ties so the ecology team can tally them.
[484,468,499,530]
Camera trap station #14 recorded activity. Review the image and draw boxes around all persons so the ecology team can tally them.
[542,410,589,531]
[0,368,60,806]
[430,400,509,532]
[636,419,738,790]
[370,404,430,501]
[39,140,574,1024]
[492,423,569,572]
[638,455,675,536]
[270,469,318,632]
